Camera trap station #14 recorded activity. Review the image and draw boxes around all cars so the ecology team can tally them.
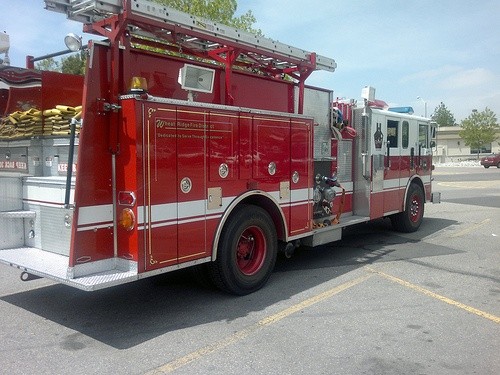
[481,154,500,168]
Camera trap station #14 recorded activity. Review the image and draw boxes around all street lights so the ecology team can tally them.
[416,95,427,118]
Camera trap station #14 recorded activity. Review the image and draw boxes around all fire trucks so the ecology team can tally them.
[0,0,441,296]
